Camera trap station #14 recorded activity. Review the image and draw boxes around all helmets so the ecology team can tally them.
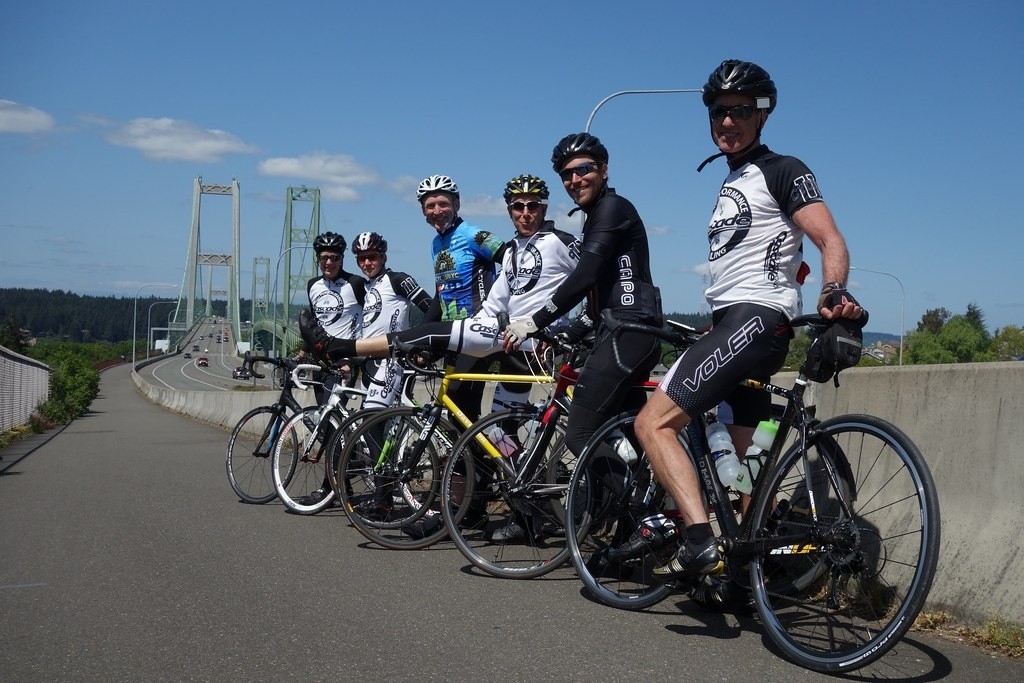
[551,133,608,173]
[352,232,388,255]
[416,176,459,202]
[703,60,777,114]
[313,232,347,253]
[504,174,549,204]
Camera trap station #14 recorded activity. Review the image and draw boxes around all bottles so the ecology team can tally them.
[734,418,780,496]
[608,430,637,465]
[520,398,548,450]
[478,415,518,458]
[705,413,741,488]
[351,407,365,434]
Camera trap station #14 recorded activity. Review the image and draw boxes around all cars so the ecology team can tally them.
[197,358,210,367]
[193,345,199,351]
[208,318,229,343]
[232,366,250,380]
[199,336,203,340]
[183,352,191,358]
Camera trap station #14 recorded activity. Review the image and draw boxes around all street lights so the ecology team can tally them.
[146,301,178,361]
[272,246,349,389]
[849,266,904,365]
[132,284,179,370]
[167,309,191,336]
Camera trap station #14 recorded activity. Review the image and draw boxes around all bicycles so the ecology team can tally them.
[227,339,667,567]
[438,326,857,614]
[563,308,942,676]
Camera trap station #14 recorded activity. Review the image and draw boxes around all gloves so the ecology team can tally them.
[292,354,308,364]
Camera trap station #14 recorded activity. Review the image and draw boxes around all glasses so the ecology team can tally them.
[510,201,540,211]
[708,104,762,121]
[355,252,383,262]
[558,162,597,181]
[315,254,343,263]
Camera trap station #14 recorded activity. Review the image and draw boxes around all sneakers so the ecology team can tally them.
[609,515,679,564]
[351,499,394,521]
[693,574,784,611]
[299,308,337,371]
[298,488,344,507]
[652,539,719,581]
[459,510,489,531]
[484,523,545,545]
[402,515,462,541]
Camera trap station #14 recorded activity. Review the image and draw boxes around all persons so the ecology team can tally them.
[351,232,433,520]
[280,232,369,507]
[298,132,666,558]
[399,174,505,540]
[634,58,863,607]
